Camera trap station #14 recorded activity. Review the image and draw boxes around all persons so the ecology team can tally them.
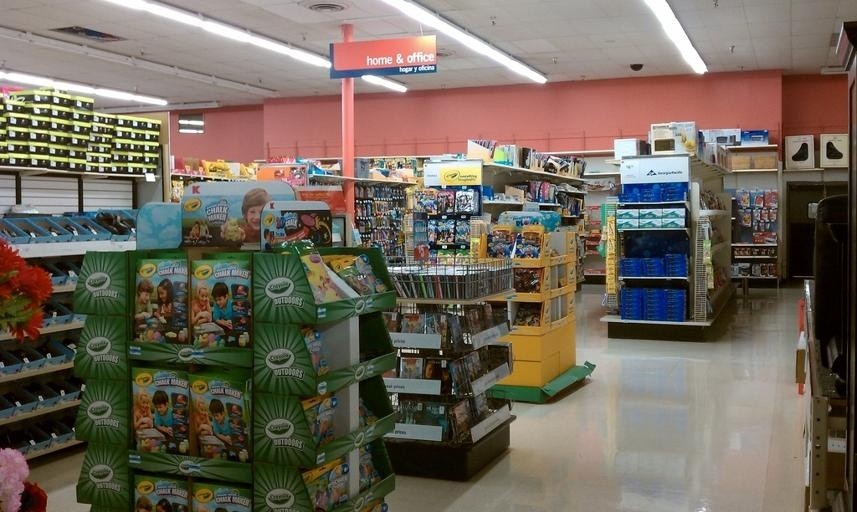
[133,389,151,429]
[214,283,238,331]
[156,279,173,331]
[193,399,213,435]
[135,495,153,512]
[220,187,272,244]
[190,283,212,325]
[151,391,175,437]
[214,399,231,447]
[136,276,152,327]
[155,497,173,512]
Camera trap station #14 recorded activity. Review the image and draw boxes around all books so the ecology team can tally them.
[470,140,586,217]
[381,263,516,442]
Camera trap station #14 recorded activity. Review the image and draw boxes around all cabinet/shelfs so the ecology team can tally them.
[798,277,856,512]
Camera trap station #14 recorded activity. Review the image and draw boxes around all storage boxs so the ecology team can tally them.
[0,84,161,177]
